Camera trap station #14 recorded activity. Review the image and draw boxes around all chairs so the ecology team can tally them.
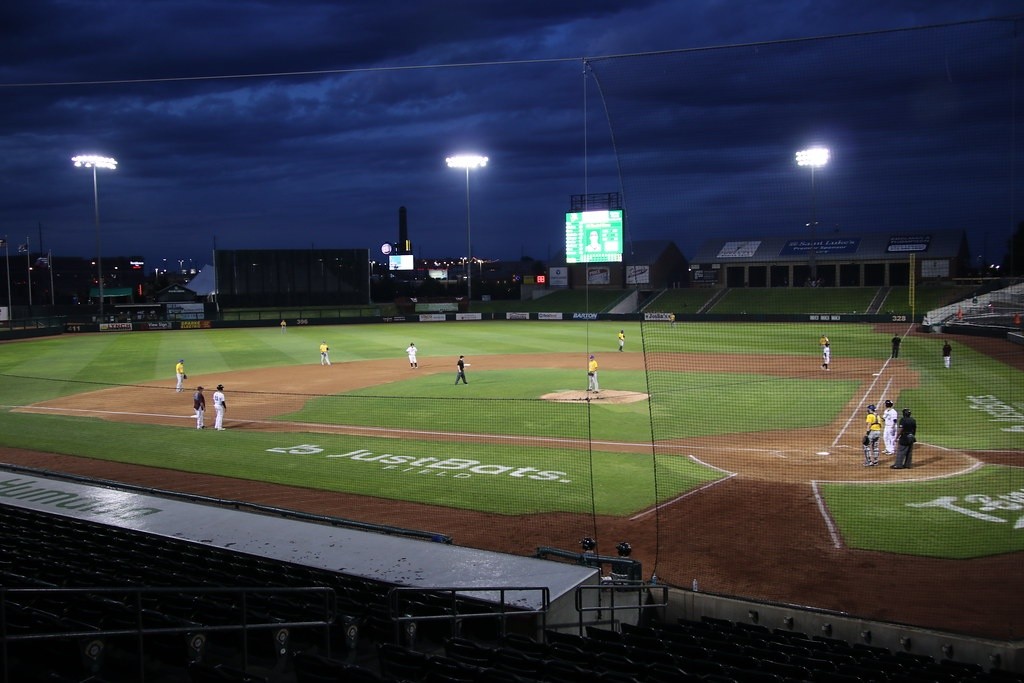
[0,503,1024,683]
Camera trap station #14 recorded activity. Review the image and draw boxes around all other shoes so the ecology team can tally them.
[890,465,901,469]
[881,449,896,455]
[863,461,879,466]
[215,427,226,431]
[593,391,599,393]
[586,389,592,391]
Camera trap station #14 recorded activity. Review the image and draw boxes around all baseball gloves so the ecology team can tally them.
[184,374,187,379]
[862,436,870,446]
[587,372,594,376]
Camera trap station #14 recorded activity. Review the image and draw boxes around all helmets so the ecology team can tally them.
[902,408,911,416]
[217,384,224,390]
[579,537,596,550]
[615,542,632,556]
[885,400,894,407]
[866,405,876,412]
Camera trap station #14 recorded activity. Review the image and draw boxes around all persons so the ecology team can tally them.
[193,387,207,431]
[890,408,917,471]
[213,384,227,431]
[819,335,831,371]
[670,312,675,327]
[942,339,952,368]
[578,537,632,575]
[586,354,599,393]
[406,342,418,369]
[175,360,187,393]
[862,405,884,467]
[618,330,625,352]
[455,356,467,385]
[892,334,901,358]
[280,319,287,334]
[320,341,331,366]
[881,400,898,456]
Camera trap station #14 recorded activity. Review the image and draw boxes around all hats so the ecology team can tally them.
[589,355,594,359]
[197,386,204,390]
